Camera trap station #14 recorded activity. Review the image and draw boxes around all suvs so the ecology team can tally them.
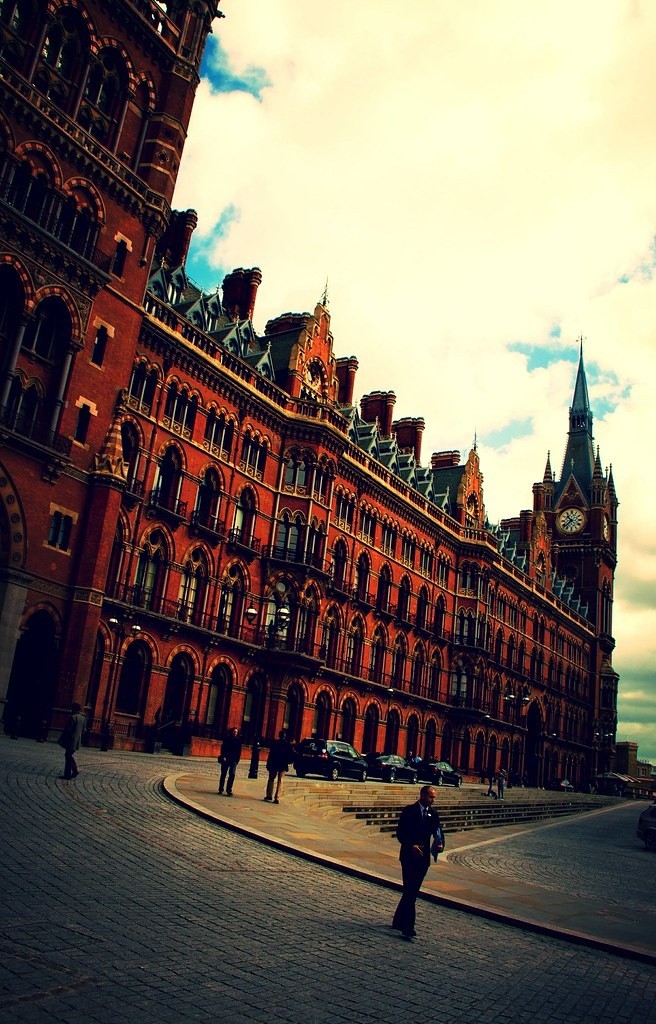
[293,737,369,782]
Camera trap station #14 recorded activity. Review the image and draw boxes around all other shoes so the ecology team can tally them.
[392,918,417,938]
[218,791,234,796]
[59,772,80,779]
[264,796,280,803]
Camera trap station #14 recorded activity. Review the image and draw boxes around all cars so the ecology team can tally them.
[363,752,419,784]
[547,778,573,792]
[636,799,655,851]
[413,759,463,787]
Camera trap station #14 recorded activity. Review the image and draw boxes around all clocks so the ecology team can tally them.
[556,505,587,537]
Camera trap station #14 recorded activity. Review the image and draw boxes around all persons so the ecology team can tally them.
[392,786,446,936]
[414,753,423,763]
[264,730,293,804]
[488,764,496,795]
[406,751,416,765]
[497,765,507,800]
[219,728,242,796]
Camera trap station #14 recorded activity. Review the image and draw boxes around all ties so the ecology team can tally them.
[422,808,427,817]
